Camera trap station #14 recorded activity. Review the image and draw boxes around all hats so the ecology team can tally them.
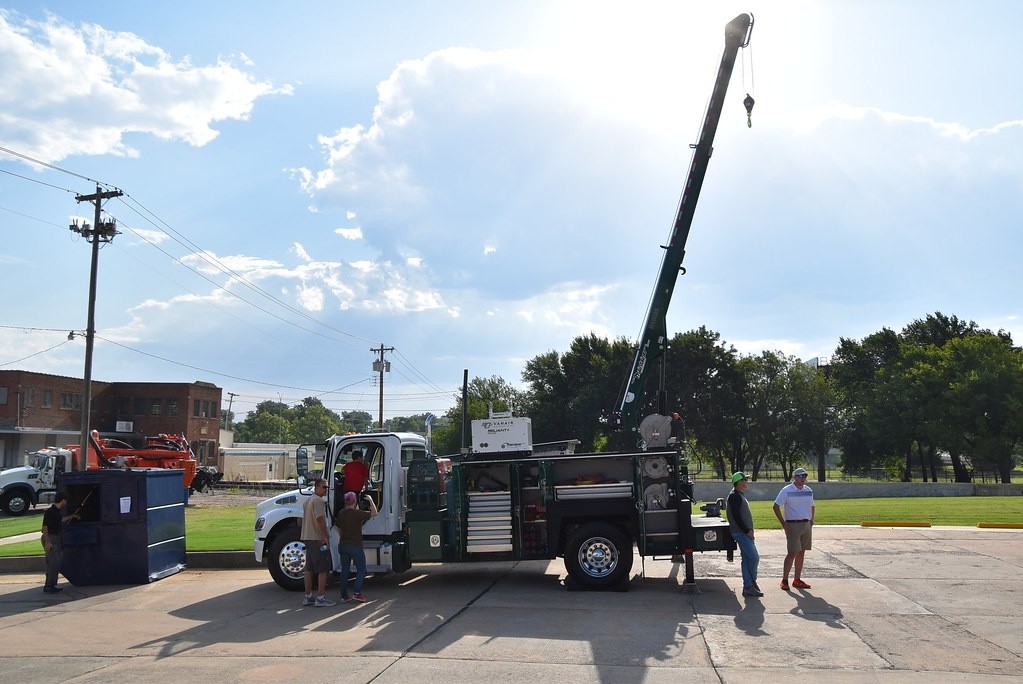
[344,492,356,504]
[793,468,808,477]
[731,472,750,484]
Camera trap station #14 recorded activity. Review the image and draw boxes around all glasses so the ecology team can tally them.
[745,478,748,481]
[318,484,327,489]
[796,475,807,479]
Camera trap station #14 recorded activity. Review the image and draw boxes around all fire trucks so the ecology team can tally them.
[0,427,200,517]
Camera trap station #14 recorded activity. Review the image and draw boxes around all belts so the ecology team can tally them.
[786,519,808,522]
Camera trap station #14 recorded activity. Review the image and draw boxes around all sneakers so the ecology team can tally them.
[303,595,316,605]
[340,595,356,604]
[351,593,368,601]
[792,579,810,588]
[314,596,336,606]
[780,579,790,590]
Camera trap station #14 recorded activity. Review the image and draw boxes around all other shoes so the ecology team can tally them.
[44,586,64,593]
[742,581,764,597]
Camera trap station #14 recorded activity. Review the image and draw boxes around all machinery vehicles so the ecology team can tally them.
[252,12,758,592]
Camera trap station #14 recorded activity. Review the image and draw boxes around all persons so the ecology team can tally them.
[40,492,77,594]
[342,451,369,494]
[773,468,816,589]
[335,491,377,602]
[726,471,764,596]
[299,478,336,606]
